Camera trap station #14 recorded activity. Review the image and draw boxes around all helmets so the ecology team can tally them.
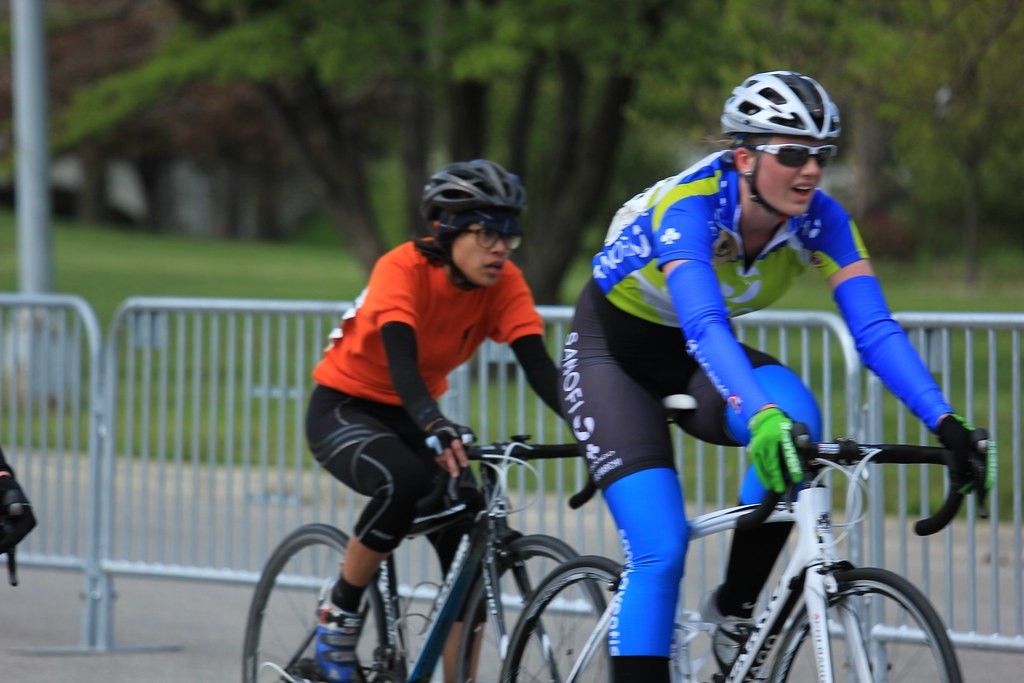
[721,71,842,139]
[420,160,524,220]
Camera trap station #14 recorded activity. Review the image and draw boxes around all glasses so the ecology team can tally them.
[735,143,837,168]
[462,227,523,250]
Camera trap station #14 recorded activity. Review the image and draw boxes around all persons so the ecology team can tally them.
[302,158,561,683]
[563,72,997,683]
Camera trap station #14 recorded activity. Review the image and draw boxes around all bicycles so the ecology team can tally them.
[241,435,615,683]
[500,422,989,683]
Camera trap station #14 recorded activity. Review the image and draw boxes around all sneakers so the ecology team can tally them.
[315,578,370,683]
[699,583,757,676]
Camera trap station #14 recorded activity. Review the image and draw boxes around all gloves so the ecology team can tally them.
[936,413,999,520]
[746,407,808,493]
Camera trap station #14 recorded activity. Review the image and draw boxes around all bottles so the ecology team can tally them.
[0,464,25,520]
